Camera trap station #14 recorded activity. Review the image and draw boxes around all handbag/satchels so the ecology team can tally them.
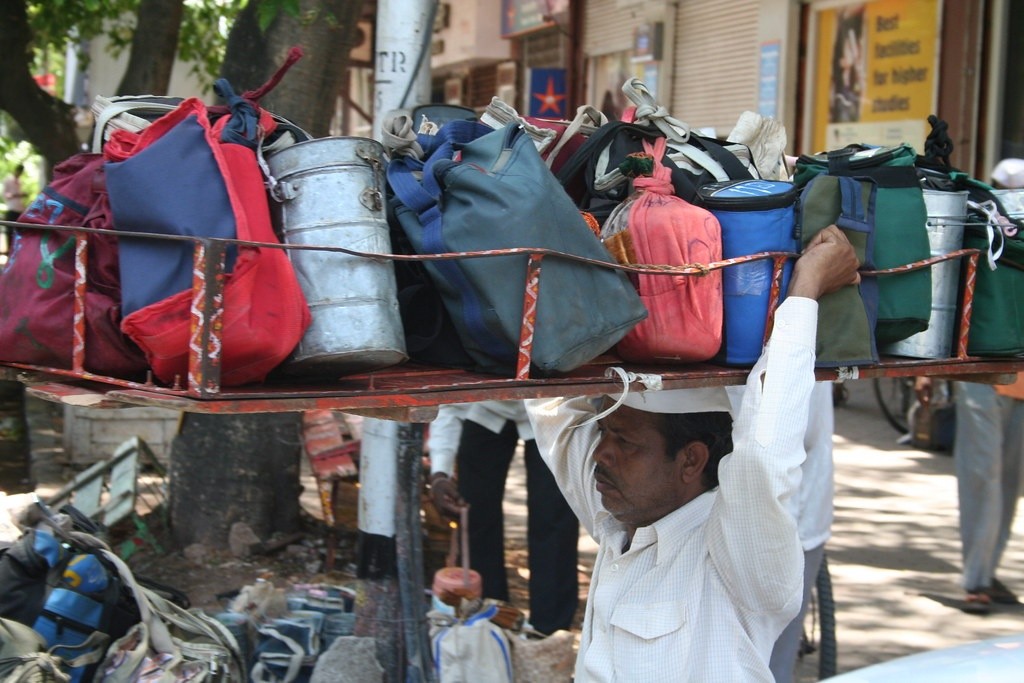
[696,178,801,368]
[425,606,513,683]
[598,136,723,366]
[0,152,144,376]
[950,170,1024,357]
[794,142,932,365]
[554,77,763,227]
[88,93,315,157]
[0,491,357,683]
[478,95,609,175]
[104,98,312,388]
[386,119,649,377]
[430,506,483,617]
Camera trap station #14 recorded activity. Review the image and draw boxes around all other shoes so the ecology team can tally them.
[960,588,992,612]
[992,578,1017,604]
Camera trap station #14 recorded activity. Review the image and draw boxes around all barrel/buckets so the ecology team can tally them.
[878,186,970,360]
[257,136,409,368]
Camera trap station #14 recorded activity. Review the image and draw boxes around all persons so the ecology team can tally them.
[3,165,30,253]
[914,158,1024,611]
[428,378,580,640]
[523,224,862,683]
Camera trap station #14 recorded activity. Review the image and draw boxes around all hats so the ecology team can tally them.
[990,157,1024,188]
[606,388,733,414]
